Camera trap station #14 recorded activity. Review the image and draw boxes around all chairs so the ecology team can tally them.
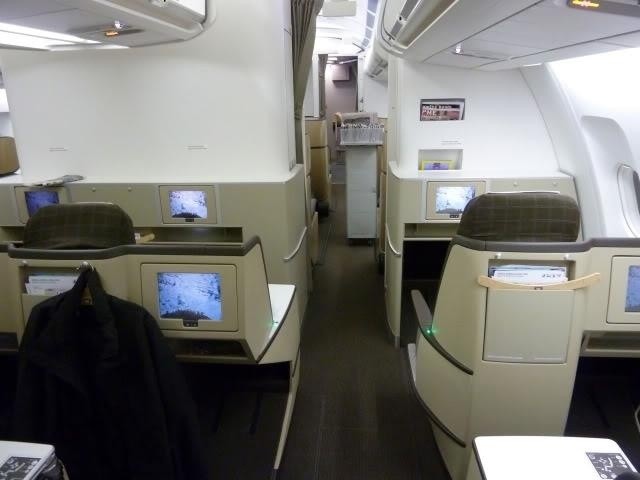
[405,192,586,479]
[0,202,137,354]
[135,235,300,469]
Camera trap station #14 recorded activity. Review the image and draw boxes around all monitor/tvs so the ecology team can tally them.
[141,263,238,332]
[426,181,486,220]
[15,186,69,223]
[159,185,217,224]
[606,256,640,324]
